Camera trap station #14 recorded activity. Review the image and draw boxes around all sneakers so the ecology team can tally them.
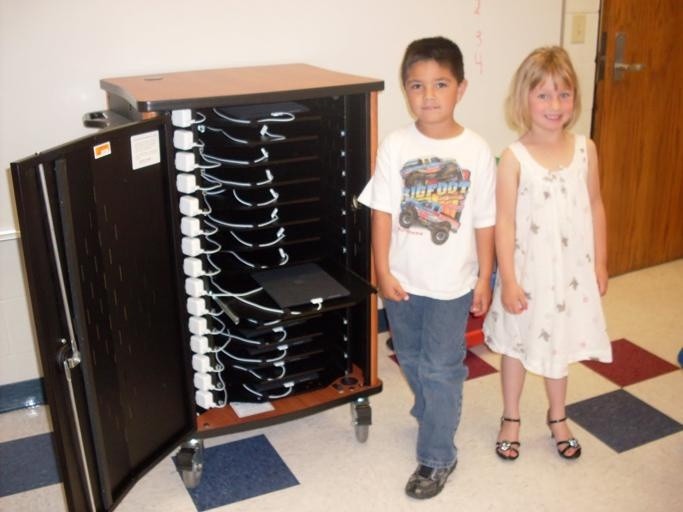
[405,458,458,499]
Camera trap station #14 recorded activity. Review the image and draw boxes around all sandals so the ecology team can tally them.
[495,416,521,460]
[547,409,582,460]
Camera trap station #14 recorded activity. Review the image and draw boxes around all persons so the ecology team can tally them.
[479,44,612,460]
[355,33,499,501]
